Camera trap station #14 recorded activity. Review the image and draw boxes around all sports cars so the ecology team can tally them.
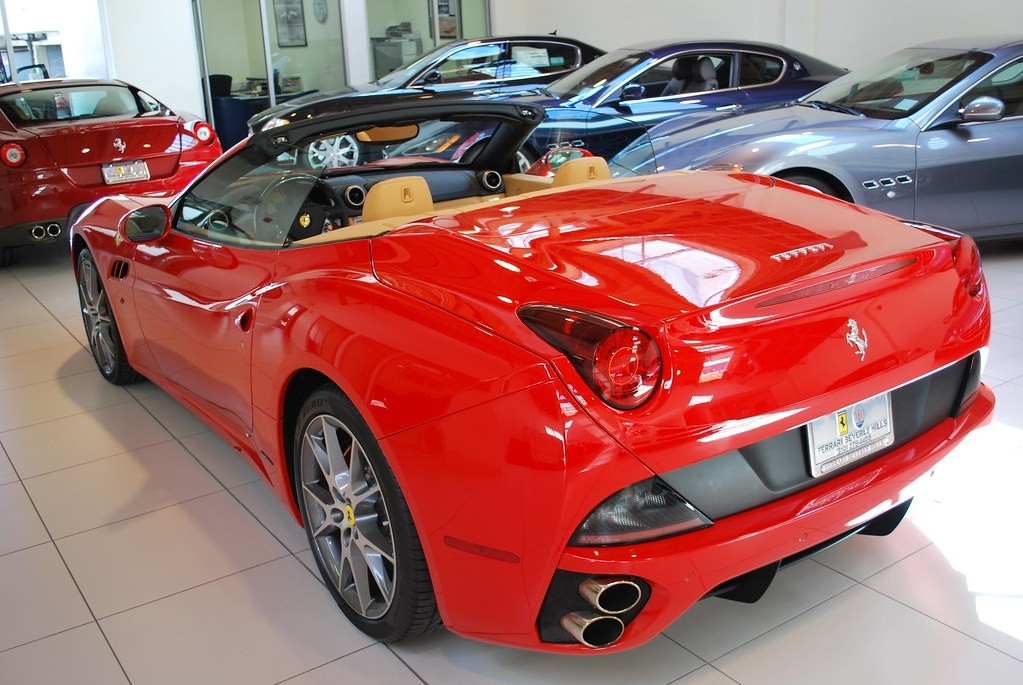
[70,95,997,654]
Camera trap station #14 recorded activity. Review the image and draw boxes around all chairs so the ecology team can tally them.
[660,57,697,95]
[684,57,718,93]
[550,156,610,188]
[362,175,434,221]
[22,92,59,120]
[93,96,129,115]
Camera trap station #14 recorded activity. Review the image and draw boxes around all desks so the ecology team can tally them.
[374,38,422,80]
[216,89,319,153]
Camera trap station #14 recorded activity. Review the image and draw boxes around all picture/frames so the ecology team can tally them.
[273,0,307,48]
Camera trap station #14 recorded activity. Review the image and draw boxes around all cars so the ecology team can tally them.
[54,92,70,109]
[247,30,605,171]
[383,39,855,173]
[0,77,224,268]
[607,35,1023,244]
